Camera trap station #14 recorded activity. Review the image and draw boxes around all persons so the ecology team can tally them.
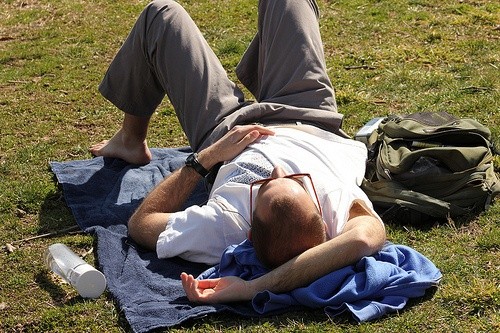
[88,0,387,303]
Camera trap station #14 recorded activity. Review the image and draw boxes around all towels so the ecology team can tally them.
[42,147,269,332]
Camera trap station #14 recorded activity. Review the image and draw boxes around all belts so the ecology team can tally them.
[250,121,328,130]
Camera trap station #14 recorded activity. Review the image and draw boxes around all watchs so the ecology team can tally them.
[185,153,210,179]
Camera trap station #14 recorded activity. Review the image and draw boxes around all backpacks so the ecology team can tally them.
[362,111,500,217]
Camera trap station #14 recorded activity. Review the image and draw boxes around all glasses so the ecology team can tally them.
[250,173,322,225]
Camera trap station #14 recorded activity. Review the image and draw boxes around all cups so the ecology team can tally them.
[43,243,106,297]
